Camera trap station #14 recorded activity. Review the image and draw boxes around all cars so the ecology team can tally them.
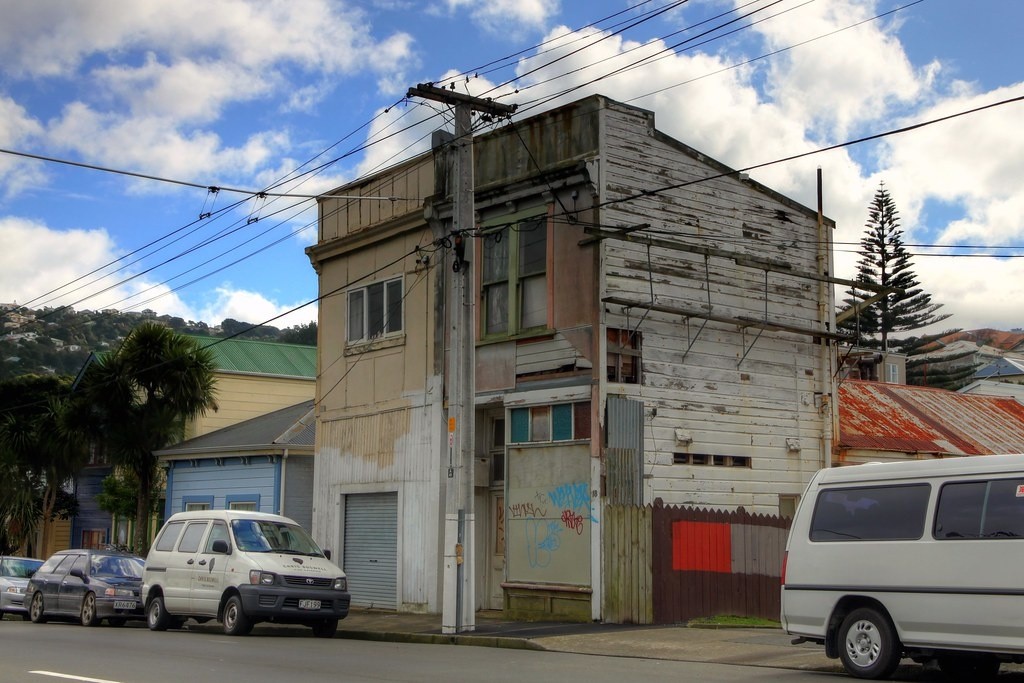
[0,556,47,621]
[23,548,146,628]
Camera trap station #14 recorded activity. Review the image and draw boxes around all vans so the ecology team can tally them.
[142,508,351,635]
[778,453,1023,679]
